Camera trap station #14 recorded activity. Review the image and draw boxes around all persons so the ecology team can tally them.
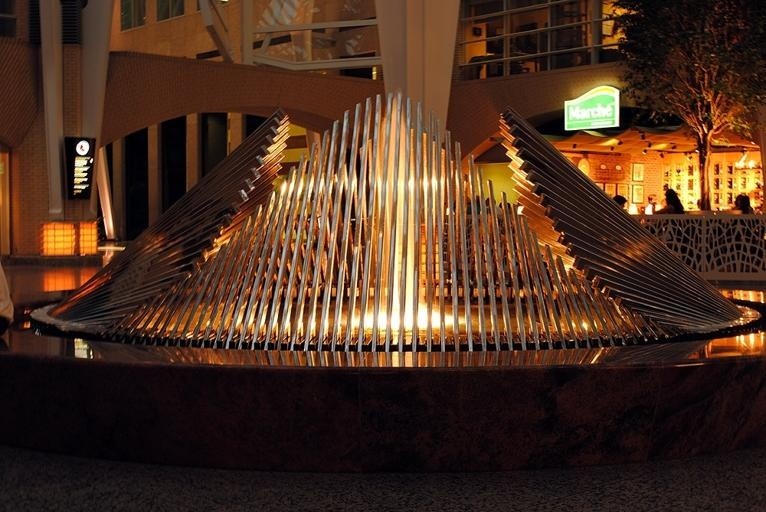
[737,196,753,215]
[0,263,14,337]
[653,190,684,214]
[732,195,744,210]
[613,196,627,209]
[645,194,664,215]
